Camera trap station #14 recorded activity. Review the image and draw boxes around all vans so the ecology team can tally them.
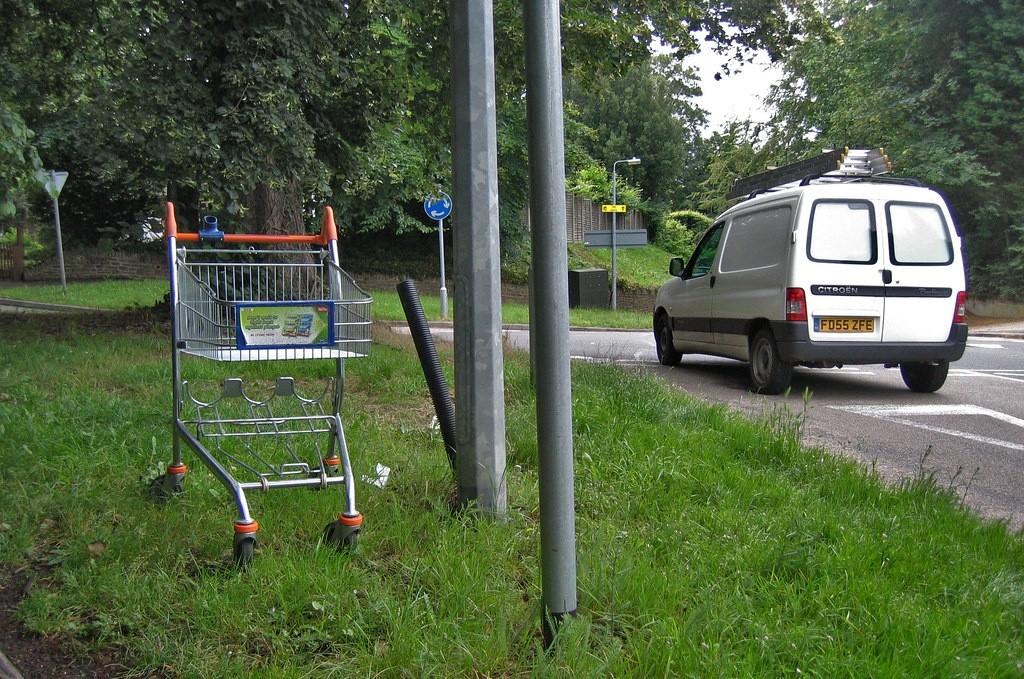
[652,144,969,395]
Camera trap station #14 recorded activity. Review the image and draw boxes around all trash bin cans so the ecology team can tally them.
[568,268,610,309]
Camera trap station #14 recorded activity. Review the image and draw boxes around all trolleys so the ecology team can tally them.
[146,201,365,574]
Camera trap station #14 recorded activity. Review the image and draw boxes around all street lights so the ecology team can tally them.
[611,157,642,310]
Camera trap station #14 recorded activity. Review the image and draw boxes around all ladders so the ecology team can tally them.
[724,145,892,202]
